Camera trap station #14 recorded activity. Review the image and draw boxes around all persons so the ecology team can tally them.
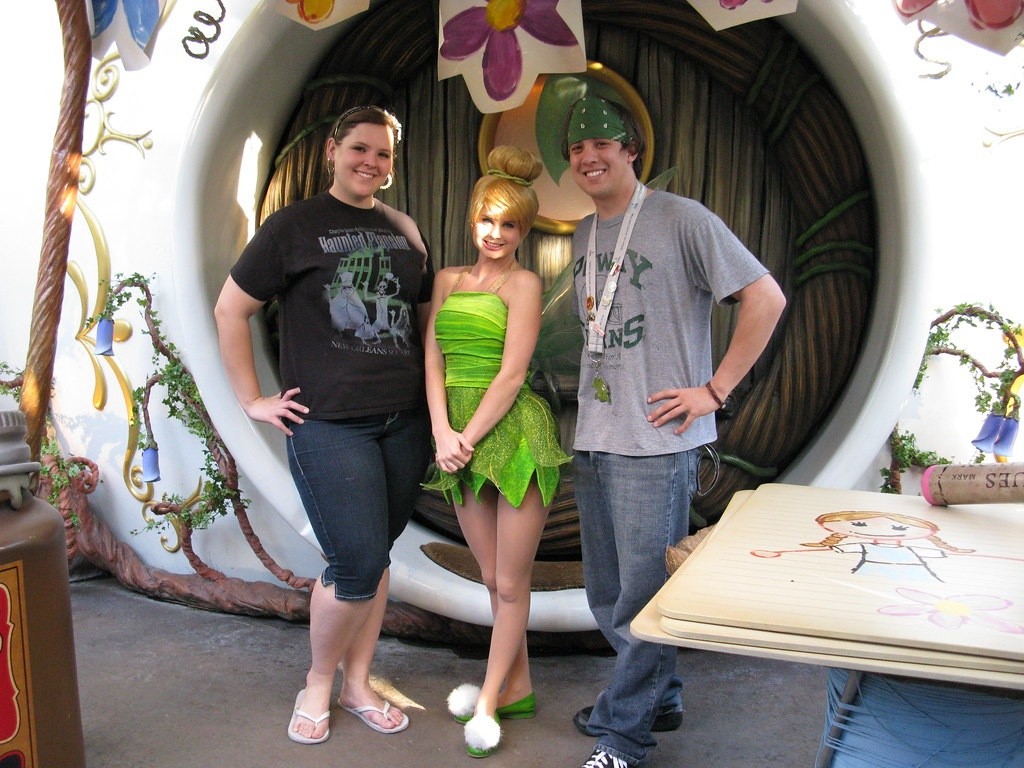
[424,144,574,758]
[214,104,436,745]
[561,92,788,768]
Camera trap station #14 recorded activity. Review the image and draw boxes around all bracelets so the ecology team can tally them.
[706,381,727,409]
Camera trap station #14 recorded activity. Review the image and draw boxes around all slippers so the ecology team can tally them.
[287,689,330,744]
[337,696,410,733]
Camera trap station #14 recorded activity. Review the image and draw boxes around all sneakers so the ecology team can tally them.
[579,748,632,768]
[573,703,682,737]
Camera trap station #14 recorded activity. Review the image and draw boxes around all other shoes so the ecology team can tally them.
[447,684,536,725]
[463,710,501,758]
